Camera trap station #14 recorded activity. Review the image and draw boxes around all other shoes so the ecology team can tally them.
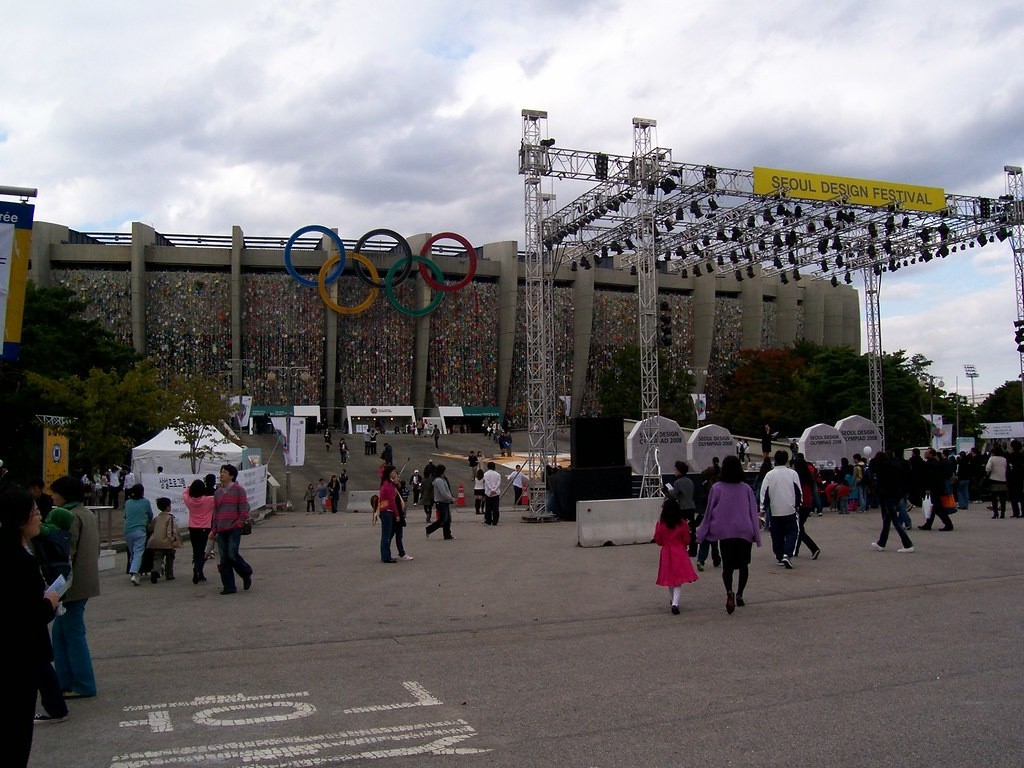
[777,560,784,565]
[219,588,237,595]
[131,575,141,585]
[782,554,794,569]
[34,711,68,725]
[444,536,456,541]
[426,526,430,537]
[685,506,1024,558]
[383,560,397,563]
[243,569,253,590]
[670,600,680,616]
[810,549,821,560]
[166,576,176,580]
[151,570,158,584]
[696,561,704,572]
[62,689,92,699]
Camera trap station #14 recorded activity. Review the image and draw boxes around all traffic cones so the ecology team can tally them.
[520,482,529,505]
[455,482,466,507]
[325,498,332,509]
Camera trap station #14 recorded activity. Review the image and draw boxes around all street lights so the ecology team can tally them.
[918,372,945,449]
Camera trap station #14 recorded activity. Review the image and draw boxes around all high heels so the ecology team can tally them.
[725,590,736,615]
[736,594,745,607]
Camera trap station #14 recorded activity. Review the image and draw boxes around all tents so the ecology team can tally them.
[131,396,243,474]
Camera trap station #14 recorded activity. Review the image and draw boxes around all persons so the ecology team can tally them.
[670,456,722,571]
[761,424,774,459]
[793,440,1024,559]
[760,449,803,568]
[507,465,529,505]
[468,450,501,526]
[394,417,440,449]
[124,463,253,595]
[364,427,393,465]
[316,418,349,465]
[303,469,348,515]
[0,474,100,768]
[654,496,699,615]
[483,417,513,457]
[379,459,457,562]
[736,439,751,462]
[83,463,135,509]
[696,455,763,616]
[790,439,798,467]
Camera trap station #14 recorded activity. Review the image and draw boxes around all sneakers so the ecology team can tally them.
[391,554,398,559]
[400,554,413,560]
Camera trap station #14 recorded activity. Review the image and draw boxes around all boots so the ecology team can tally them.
[191,557,209,585]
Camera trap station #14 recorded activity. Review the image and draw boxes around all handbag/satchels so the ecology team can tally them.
[239,522,252,535]
[923,494,932,518]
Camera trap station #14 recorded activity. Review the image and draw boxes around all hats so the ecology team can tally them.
[49,475,87,503]
[414,470,419,474]
